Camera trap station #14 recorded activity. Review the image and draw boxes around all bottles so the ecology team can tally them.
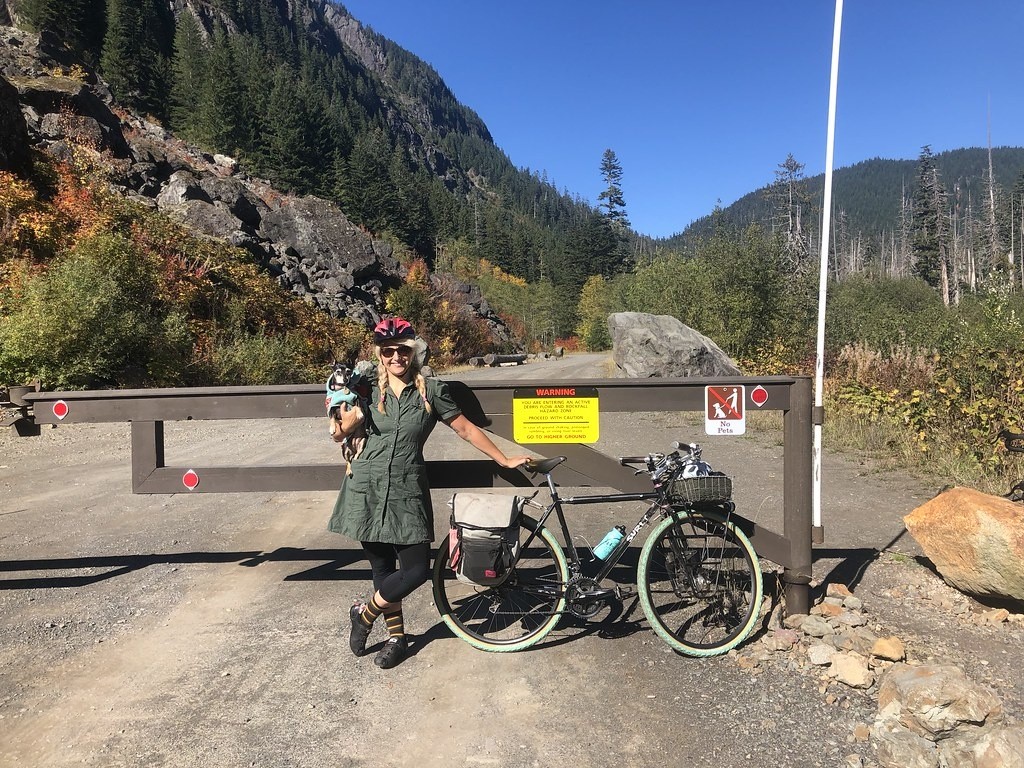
[591,525,626,563]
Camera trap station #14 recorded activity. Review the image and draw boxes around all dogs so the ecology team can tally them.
[325,349,368,479]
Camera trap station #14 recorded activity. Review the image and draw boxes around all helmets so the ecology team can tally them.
[372,316,415,343]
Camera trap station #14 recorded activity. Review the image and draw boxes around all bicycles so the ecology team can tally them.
[431,440,764,658]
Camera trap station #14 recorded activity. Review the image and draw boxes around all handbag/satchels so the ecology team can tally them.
[448,492,525,587]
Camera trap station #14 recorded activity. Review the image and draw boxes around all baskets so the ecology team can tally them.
[670,475,732,505]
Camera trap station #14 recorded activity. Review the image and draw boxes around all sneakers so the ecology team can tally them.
[374,634,407,668]
[350,602,371,655]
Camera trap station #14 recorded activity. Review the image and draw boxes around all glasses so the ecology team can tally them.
[381,345,414,357]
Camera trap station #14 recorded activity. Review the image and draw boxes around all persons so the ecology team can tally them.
[331,317,532,668]
